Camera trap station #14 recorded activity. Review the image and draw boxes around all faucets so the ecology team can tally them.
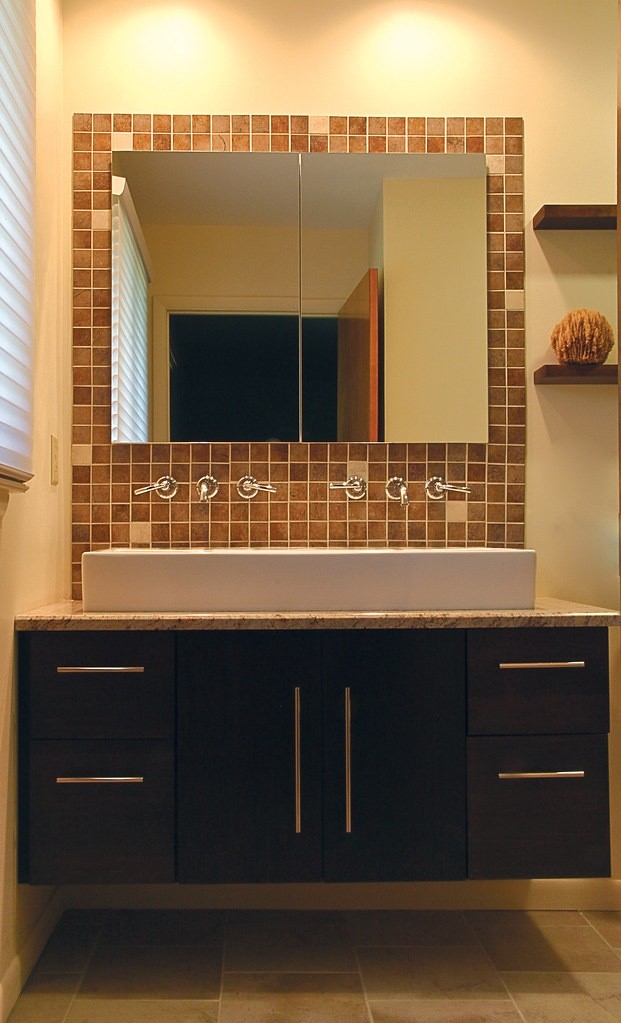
[384,475,409,505]
[196,474,219,503]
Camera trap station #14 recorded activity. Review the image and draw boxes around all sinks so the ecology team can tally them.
[80,546,538,614]
